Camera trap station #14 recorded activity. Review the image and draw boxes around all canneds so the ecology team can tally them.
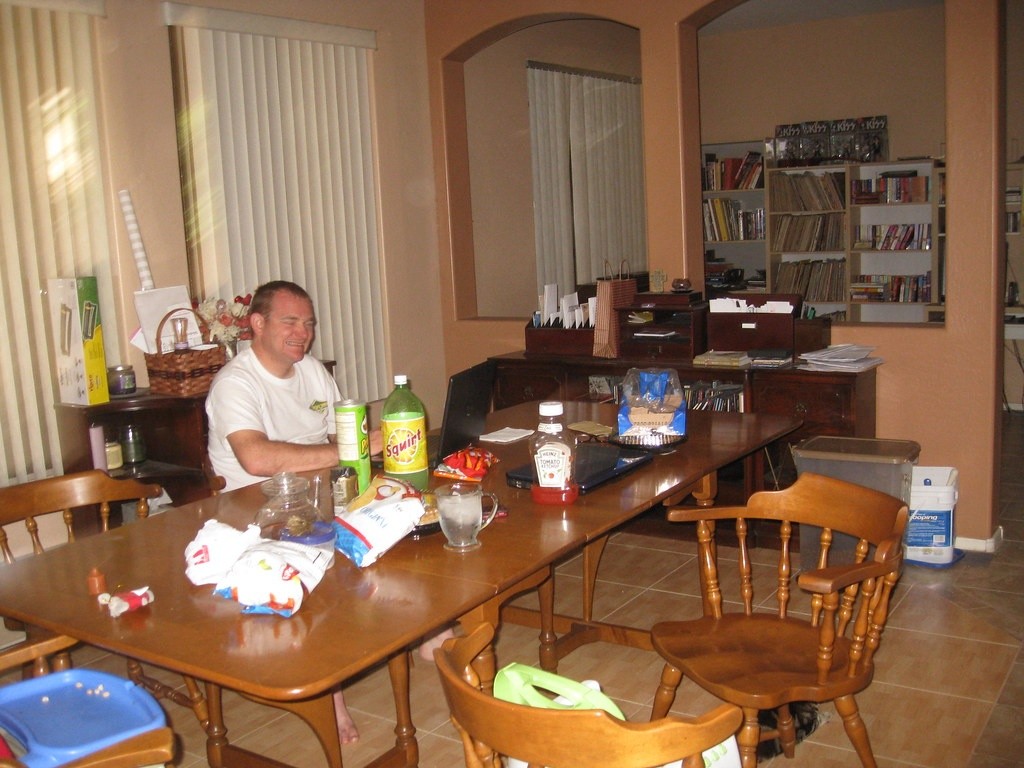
[107,365,136,395]
[334,399,371,497]
[330,466,359,518]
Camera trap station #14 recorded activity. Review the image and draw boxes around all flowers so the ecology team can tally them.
[192,295,253,357]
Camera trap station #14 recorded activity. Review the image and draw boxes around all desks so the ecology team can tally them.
[0,398,803,768]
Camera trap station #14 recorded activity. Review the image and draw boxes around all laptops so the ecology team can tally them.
[507,444,657,494]
[369,355,499,470]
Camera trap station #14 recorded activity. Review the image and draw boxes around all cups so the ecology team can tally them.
[434,482,499,553]
[1006,212,1018,232]
[278,521,336,570]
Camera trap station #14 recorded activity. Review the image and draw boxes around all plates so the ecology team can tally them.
[108,387,150,398]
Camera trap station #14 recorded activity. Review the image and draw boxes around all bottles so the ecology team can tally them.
[528,402,578,504]
[1008,282,1018,305]
[88,416,147,473]
[89,568,106,593]
[382,375,429,491]
[107,365,136,394]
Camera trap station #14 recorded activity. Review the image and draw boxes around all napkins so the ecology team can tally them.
[479,427,535,443]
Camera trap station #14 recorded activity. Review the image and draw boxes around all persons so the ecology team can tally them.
[204,280,458,745]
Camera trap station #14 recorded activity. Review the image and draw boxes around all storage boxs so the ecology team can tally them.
[46,276,109,406]
[706,293,831,349]
[793,436,921,573]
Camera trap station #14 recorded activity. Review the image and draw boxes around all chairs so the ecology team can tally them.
[650,473,910,767]
[1,631,177,768]
[433,621,744,768]
[0,470,211,732]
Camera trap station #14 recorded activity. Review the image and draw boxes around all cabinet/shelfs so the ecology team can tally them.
[53,357,337,541]
[700,137,1024,341]
[488,349,877,554]
[614,303,709,362]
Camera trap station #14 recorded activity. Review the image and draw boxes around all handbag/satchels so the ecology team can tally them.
[593,259,637,359]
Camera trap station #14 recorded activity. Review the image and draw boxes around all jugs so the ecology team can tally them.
[253,471,326,541]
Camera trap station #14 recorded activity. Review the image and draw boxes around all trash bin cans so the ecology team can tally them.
[791,433,921,593]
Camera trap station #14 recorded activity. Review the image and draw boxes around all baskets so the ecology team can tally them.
[144,308,226,399]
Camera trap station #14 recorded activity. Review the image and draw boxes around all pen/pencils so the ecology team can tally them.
[801,304,816,320]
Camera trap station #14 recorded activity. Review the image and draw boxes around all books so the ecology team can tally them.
[770,172,846,211]
[852,171,932,203]
[797,343,885,372]
[774,213,846,251]
[752,357,792,367]
[853,223,931,250]
[1005,186,1023,206]
[702,151,765,191]
[849,271,931,302]
[703,198,767,240]
[693,350,752,366]
[679,378,744,414]
[775,257,843,302]
[576,374,625,404]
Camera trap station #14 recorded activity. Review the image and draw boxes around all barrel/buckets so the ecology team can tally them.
[901,465,958,563]
